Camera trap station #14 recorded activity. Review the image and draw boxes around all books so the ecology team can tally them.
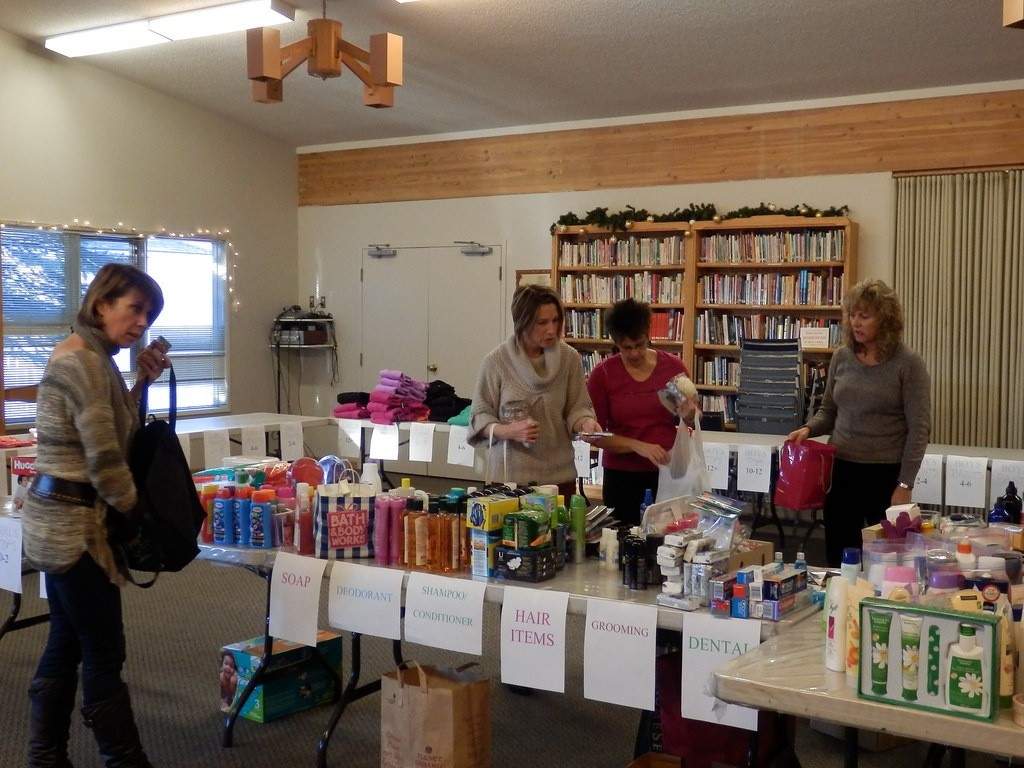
[693,229,845,424]
[558,236,684,374]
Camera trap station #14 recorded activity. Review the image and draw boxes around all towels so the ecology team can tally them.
[367,391,430,425]
[337,391,370,405]
[332,403,371,419]
[375,369,429,401]
[422,380,472,423]
[447,405,472,426]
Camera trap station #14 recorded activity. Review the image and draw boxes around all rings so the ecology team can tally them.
[153,365,158,370]
[532,424,535,427]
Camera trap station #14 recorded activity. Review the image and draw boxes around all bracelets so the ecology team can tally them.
[898,482,912,490]
[683,418,694,423]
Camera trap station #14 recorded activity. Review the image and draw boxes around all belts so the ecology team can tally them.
[30,473,94,508]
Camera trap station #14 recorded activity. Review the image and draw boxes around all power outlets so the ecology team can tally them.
[321,296,325,308]
[309,296,314,307]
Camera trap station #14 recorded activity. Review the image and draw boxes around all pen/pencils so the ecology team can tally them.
[582,432,613,436]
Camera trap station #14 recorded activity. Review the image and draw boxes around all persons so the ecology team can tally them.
[466,285,602,509]
[220,652,239,705]
[787,280,933,564]
[585,297,699,529]
[14,474,29,512]
[20,263,170,768]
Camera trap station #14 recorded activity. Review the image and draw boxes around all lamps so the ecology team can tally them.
[45,0,296,57]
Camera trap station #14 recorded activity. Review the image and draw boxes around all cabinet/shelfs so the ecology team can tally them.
[551,217,858,429]
[269,319,335,348]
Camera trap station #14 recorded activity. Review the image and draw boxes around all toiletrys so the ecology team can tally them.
[375,495,472,576]
[822,546,1015,723]
[293,482,314,556]
[199,482,295,548]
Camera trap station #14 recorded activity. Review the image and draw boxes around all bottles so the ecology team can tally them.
[598,528,619,571]
[953,542,1011,602]
[794,552,809,569]
[774,552,783,568]
[556,495,586,568]
[639,488,654,521]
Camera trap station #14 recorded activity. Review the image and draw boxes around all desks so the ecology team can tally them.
[579,430,791,548]
[775,434,1023,561]
[714,608,1024,768]
[324,416,469,492]
[148,411,328,457]
[196,537,865,768]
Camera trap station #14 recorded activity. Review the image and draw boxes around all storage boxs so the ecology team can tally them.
[809,719,917,751]
[470,529,503,577]
[859,597,1002,723]
[219,630,343,723]
[708,563,814,622]
[861,523,886,543]
[727,539,773,571]
[496,547,557,583]
[519,492,557,528]
[466,493,519,530]
[626,752,681,768]
[11,454,38,517]
[0,433,37,497]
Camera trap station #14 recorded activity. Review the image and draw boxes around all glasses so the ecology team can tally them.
[618,341,653,352]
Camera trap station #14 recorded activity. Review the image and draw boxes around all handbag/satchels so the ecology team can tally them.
[113,420,204,576]
[775,439,838,512]
[656,406,712,502]
[380,659,493,767]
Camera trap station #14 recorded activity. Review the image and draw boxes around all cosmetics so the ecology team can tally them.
[193,454,585,583]
[825,503,1023,727]
[600,490,813,621]
